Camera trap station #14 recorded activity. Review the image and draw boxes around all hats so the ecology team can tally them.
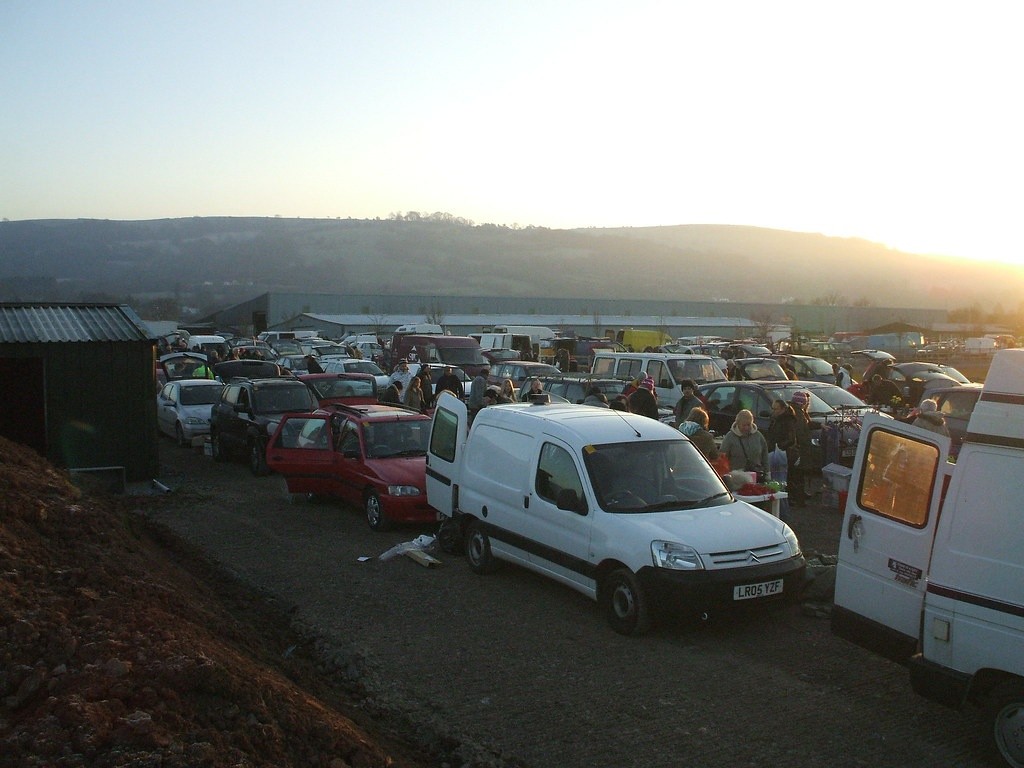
[399,358,408,365]
[792,391,807,404]
[642,376,654,391]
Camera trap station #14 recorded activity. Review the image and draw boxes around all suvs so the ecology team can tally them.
[423,392,806,637]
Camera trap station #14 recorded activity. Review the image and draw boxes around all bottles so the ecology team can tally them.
[723,473,733,494]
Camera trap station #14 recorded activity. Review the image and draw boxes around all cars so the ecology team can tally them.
[155,324,989,476]
[266,402,434,531]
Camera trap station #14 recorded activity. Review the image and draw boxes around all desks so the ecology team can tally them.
[732,490,792,521]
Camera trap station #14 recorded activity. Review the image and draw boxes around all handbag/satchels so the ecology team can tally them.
[768,443,788,491]
[746,460,765,482]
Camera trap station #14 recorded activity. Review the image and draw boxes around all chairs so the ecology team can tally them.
[392,425,419,450]
[254,392,275,408]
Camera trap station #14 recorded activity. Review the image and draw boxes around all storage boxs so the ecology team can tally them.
[822,465,852,493]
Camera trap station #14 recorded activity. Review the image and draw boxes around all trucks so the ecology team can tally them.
[831,349,1023,768]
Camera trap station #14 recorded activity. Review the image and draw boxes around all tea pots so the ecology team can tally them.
[764,479,784,493]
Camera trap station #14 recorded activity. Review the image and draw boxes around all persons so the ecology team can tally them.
[582,372,659,420]
[304,354,324,374]
[469,367,518,423]
[278,363,294,376]
[382,357,464,411]
[628,344,634,353]
[672,348,821,509]
[316,381,335,398]
[447,329,451,335]
[882,444,909,489]
[353,346,365,360]
[794,341,808,351]
[832,361,853,390]
[348,345,355,357]
[872,373,906,405]
[913,398,951,437]
[1008,339,1018,348]
[519,349,560,369]
[155,335,266,393]
[522,378,544,402]
[381,339,385,354]
